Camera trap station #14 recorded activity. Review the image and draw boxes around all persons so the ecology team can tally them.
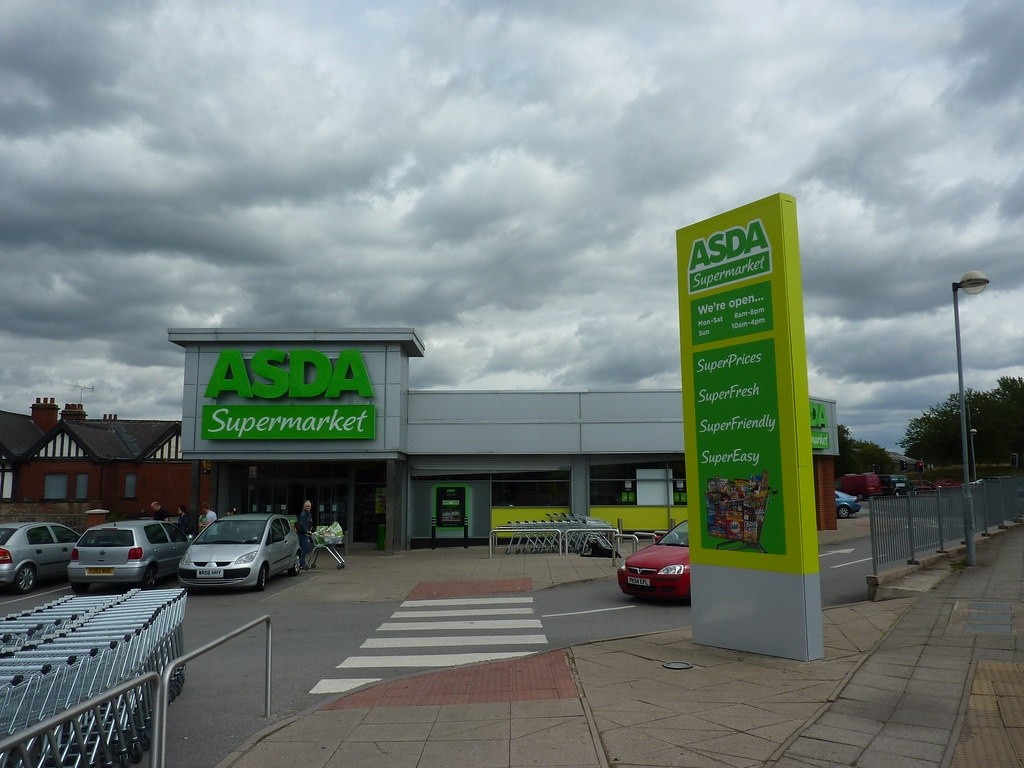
[178,505,191,535]
[198,503,217,527]
[225,507,236,516]
[296,500,313,570]
[151,502,170,522]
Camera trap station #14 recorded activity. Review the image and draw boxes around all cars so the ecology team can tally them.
[617,519,691,605]
[912,480,943,494]
[0,522,81,593]
[69,518,190,588]
[834,490,861,519]
[178,513,302,590]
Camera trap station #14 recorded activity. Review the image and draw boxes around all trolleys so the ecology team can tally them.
[304,533,346,570]
[706,488,779,554]
[502,513,614,557]
[3,587,191,767]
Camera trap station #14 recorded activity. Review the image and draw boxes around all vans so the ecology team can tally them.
[834,473,882,500]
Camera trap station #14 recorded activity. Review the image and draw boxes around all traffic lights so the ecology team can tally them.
[901,460,906,470]
[951,269,992,569]
[917,461,924,473]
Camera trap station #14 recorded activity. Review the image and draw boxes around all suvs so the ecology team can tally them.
[879,475,917,498]
